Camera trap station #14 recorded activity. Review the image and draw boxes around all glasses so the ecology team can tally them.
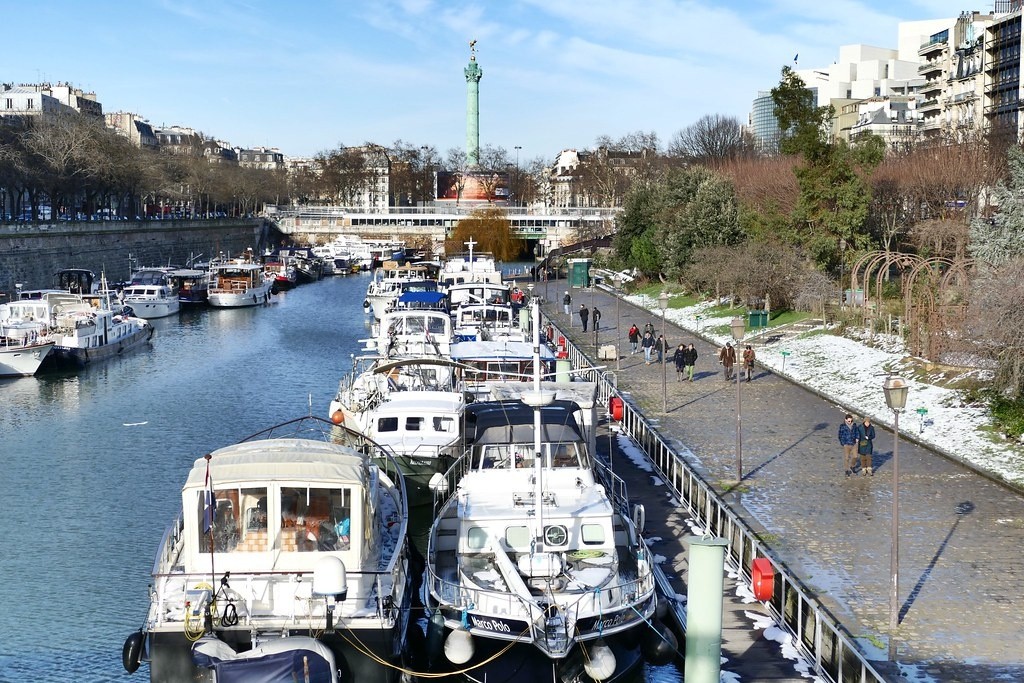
[848,421,852,422]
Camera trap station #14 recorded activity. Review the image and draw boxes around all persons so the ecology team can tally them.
[493,297,502,305]
[674,343,698,382]
[530,266,535,281]
[838,414,860,476]
[720,342,736,381]
[579,304,589,333]
[538,267,544,283]
[743,345,755,382]
[593,306,601,331]
[859,417,875,476]
[656,335,670,363]
[562,290,571,316]
[641,322,656,365]
[628,324,643,354]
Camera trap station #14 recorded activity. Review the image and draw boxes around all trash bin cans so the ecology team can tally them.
[845,289,864,306]
[747,309,768,326]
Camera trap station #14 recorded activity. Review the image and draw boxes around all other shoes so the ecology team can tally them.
[850,467,857,473]
[746,378,750,382]
[862,468,866,475]
[690,380,693,382]
[680,377,682,381]
[867,466,872,473]
[631,352,633,354]
[635,349,637,352]
[645,361,651,365]
[845,470,850,478]
[678,380,680,382]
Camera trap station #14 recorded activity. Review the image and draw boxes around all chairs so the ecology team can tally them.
[253,488,331,542]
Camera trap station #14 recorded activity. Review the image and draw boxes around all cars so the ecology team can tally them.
[0,207,227,220]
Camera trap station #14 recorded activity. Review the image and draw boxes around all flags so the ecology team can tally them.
[204,464,216,535]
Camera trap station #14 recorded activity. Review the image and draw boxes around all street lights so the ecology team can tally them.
[544,250,550,302]
[729,317,749,487]
[588,263,599,347]
[881,365,910,633]
[421,145,429,212]
[654,288,674,416]
[533,247,538,285]
[613,276,623,370]
[514,146,523,207]
[567,257,576,329]
[554,254,559,306]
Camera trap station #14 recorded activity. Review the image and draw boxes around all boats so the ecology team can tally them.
[0,229,606,564]
[123,389,416,683]
[415,299,657,683]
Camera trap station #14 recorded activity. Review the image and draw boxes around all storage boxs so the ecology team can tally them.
[229,527,307,551]
